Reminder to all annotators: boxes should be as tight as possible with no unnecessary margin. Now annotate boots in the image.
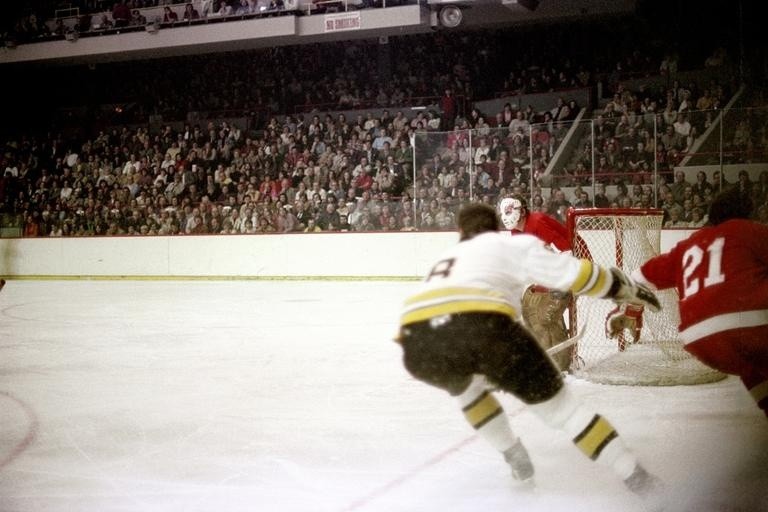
[622,464,665,499]
[502,436,535,481]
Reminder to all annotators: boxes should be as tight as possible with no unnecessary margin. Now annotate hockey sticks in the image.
[547,305,592,357]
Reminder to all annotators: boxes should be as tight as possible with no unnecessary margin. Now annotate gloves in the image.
[599,266,663,344]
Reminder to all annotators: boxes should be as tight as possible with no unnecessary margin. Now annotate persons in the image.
[602,181,767,424]
[391,201,667,503]
[1,1,767,233]
[494,188,600,375]
